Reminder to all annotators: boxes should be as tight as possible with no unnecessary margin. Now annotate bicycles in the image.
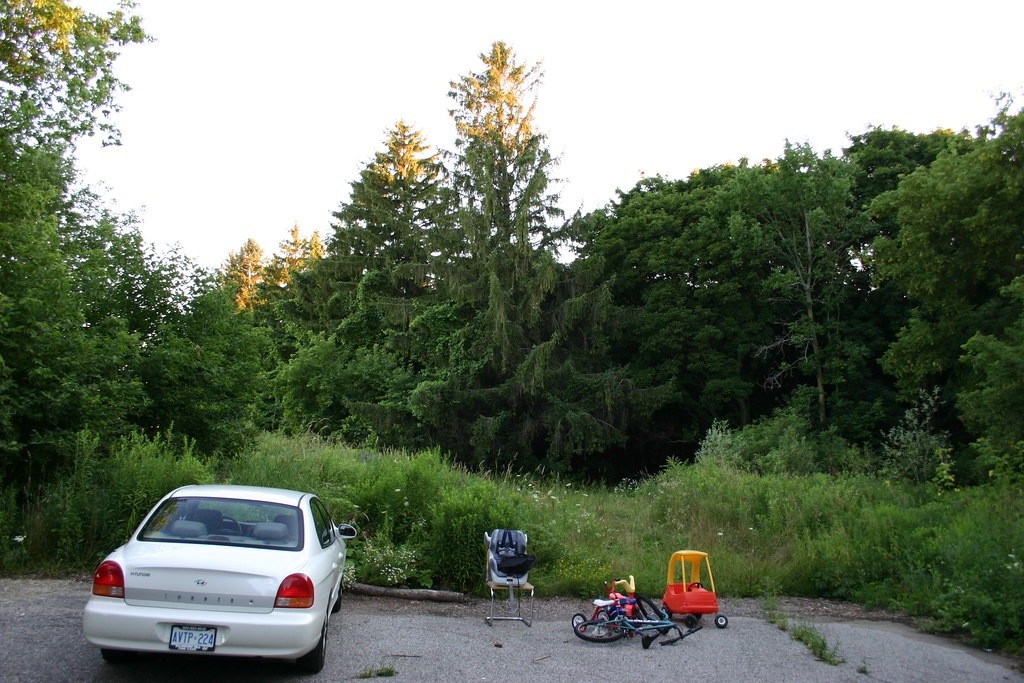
[572,574,704,649]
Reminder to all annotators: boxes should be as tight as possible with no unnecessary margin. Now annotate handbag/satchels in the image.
[496,553,537,578]
[496,529,518,557]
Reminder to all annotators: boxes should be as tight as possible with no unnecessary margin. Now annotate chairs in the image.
[171,520,207,537]
[278,515,297,537]
[196,510,223,531]
[255,521,285,541]
[485,529,534,627]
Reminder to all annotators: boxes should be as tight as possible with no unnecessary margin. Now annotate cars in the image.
[82,483,358,675]
